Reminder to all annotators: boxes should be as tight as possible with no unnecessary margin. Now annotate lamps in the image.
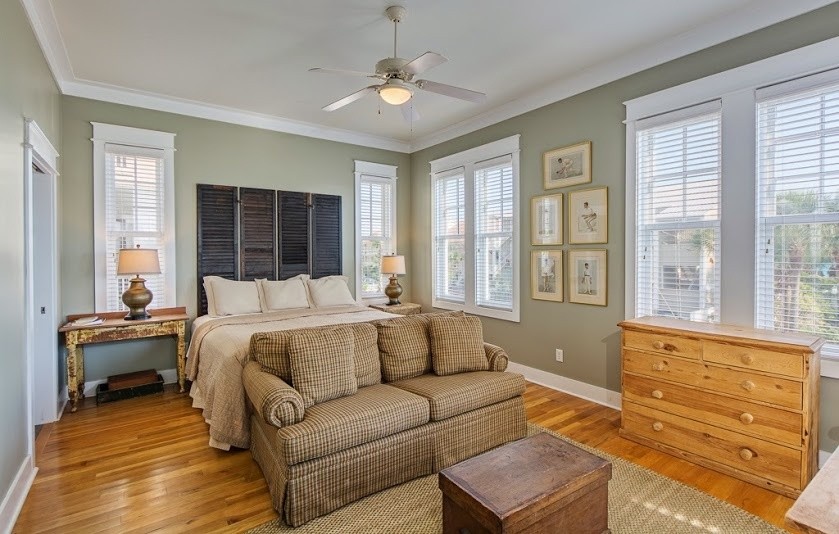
[117,244,161,320]
[380,252,406,306]
[376,80,415,137]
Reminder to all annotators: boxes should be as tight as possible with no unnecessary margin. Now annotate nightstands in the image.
[368,302,422,315]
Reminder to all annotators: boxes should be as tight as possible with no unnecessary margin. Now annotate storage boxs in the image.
[96,369,164,405]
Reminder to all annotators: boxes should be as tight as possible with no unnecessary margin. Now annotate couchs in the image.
[242,310,527,528]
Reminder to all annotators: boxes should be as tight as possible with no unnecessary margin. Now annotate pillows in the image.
[429,317,490,377]
[301,275,358,309]
[288,327,358,409]
[203,276,267,318]
[254,274,311,314]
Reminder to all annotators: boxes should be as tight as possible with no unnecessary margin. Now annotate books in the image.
[72,316,106,326]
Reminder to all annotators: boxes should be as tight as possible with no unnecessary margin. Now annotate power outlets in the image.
[556,349,563,363]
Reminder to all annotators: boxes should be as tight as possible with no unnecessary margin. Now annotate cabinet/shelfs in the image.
[616,314,826,499]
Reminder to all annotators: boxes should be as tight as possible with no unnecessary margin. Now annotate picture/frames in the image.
[543,141,592,191]
[567,248,608,307]
[530,193,564,246]
[529,249,564,303]
[568,185,608,245]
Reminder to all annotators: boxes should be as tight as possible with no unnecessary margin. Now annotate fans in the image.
[308,6,485,112]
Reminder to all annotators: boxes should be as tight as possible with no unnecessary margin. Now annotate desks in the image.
[58,307,189,413]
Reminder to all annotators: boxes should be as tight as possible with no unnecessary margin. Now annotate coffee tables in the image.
[439,432,613,534]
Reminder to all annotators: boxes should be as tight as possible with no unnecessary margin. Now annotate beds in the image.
[185,304,408,451]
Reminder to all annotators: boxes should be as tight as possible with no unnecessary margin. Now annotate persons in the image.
[555,157,574,179]
[580,200,597,232]
[541,259,552,292]
[581,263,593,294]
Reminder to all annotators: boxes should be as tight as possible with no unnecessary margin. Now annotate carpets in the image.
[243,420,793,534]
